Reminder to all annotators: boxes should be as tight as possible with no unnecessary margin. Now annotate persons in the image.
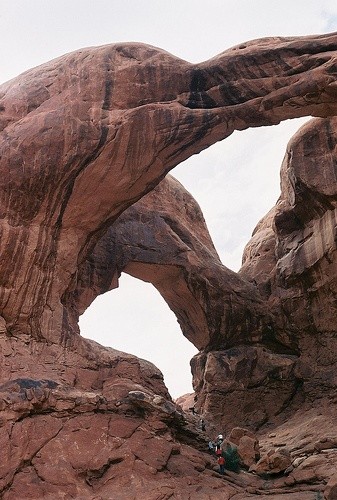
[217,432,223,442]
[253,440,263,463]
[214,442,223,473]
[227,442,239,460]
[208,437,214,455]
